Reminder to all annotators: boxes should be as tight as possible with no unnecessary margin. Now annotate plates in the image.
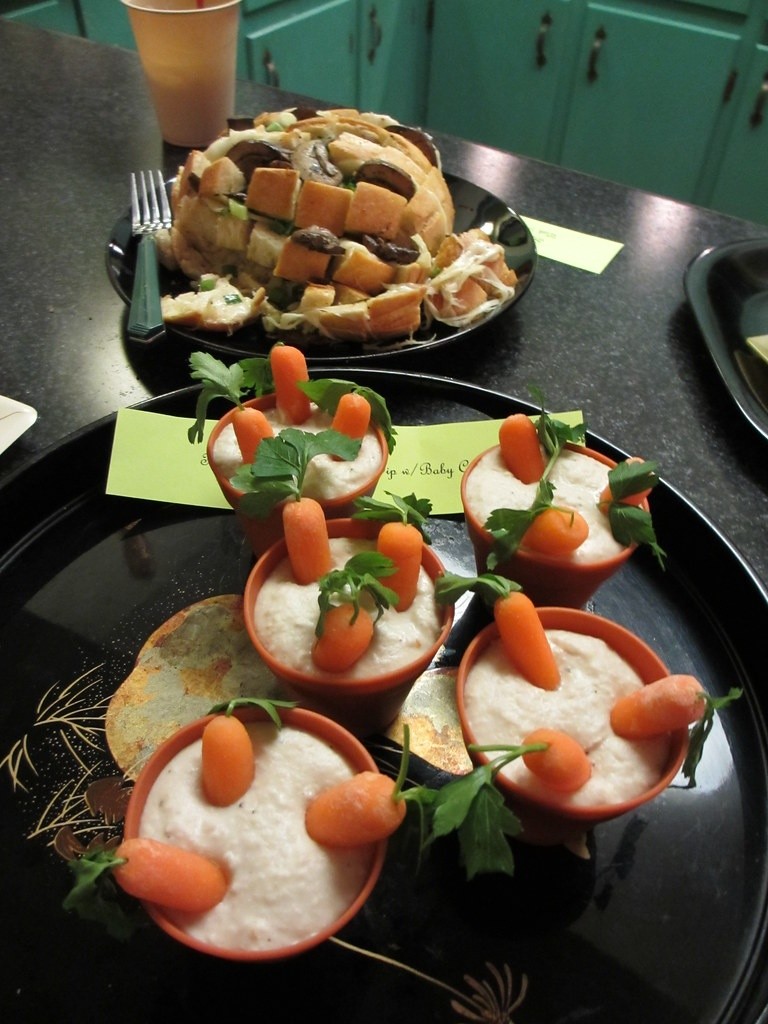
[683,235,768,441]
[105,171,538,364]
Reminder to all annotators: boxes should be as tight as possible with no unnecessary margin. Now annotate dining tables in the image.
[0,17,768,1024]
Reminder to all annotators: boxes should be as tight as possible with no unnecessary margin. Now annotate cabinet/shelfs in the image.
[424,0,752,203]
[709,0,768,225]
[240,0,430,127]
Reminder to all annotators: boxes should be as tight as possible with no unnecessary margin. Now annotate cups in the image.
[455,608,692,847]
[118,0,239,148]
[460,441,651,609]
[124,707,388,962]
[244,517,455,735]
[206,392,390,561]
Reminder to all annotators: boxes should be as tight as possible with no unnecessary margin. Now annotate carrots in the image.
[110,344,706,925]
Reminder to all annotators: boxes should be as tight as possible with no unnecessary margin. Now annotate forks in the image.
[125,173,172,342]
